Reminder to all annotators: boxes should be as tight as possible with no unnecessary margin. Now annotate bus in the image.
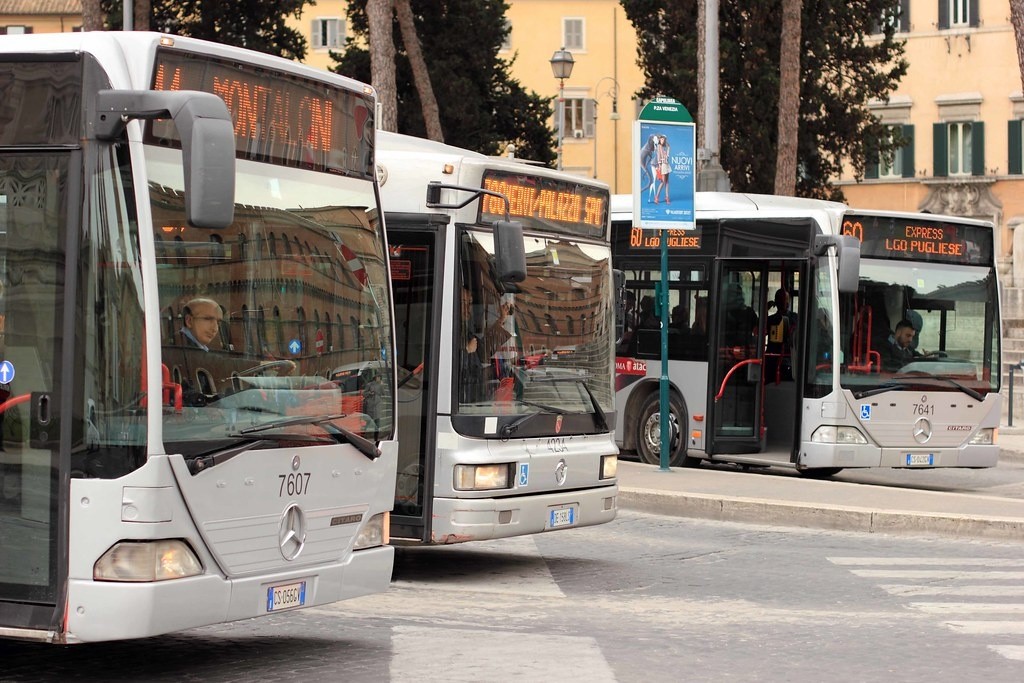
[374,130,619,549]
[610,192,1002,478]
[332,360,388,396]
[0,30,398,647]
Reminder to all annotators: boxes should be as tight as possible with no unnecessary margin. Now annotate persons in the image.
[624,290,709,355]
[875,319,931,371]
[161,299,295,389]
[647,135,671,205]
[462,290,512,380]
[906,309,924,350]
[755,288,799,373]
[726,282,759,348]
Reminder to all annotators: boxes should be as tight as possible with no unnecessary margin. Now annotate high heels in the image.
[665,196,670,204]
[655,195,658,203]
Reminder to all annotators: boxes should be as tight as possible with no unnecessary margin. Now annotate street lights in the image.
[593,77,620,178]
[551,47,574,169]
